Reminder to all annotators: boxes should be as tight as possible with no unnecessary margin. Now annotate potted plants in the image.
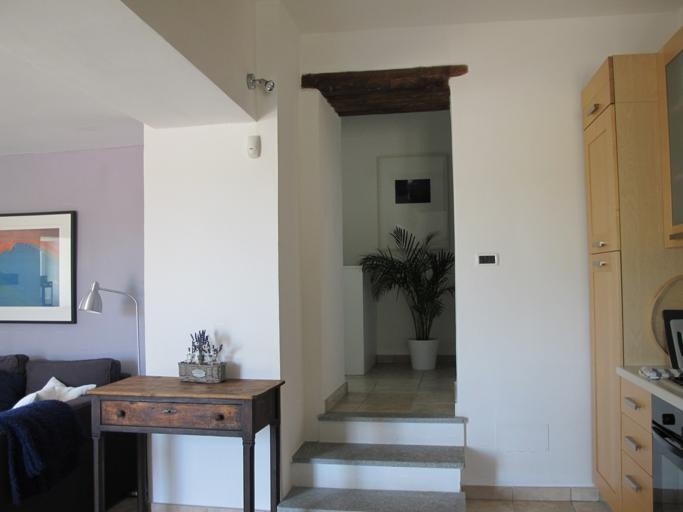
[361,224,455,372]
[177,329,229,385]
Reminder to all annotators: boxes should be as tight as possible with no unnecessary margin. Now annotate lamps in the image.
[80,276,144,377]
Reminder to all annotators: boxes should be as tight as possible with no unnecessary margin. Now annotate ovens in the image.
[652,425,683,512]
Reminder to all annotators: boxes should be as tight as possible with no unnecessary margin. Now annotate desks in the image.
[82,371,287,512]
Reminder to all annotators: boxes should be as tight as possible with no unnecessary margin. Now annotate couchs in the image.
[1,353,135,512]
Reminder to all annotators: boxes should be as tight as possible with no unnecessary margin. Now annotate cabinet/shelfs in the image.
[577,45,683,509]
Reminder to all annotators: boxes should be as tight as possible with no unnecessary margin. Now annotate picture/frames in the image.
[1,209,79,325]
